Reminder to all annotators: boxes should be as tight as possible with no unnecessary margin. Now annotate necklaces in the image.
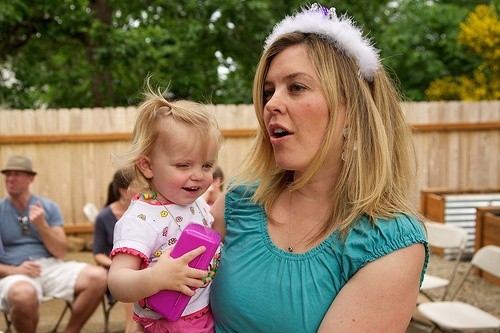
[287,188,327,253]
[142,192,217,283]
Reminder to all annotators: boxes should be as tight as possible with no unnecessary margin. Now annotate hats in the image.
[0,155,36,176]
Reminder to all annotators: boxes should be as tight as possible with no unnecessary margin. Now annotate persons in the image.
[94,167,145,333]
[108,71,224,333]
[0,156,108,333]
[210,2,429,333]
[202,167,224,205]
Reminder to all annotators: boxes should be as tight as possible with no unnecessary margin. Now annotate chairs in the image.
[0,291,118,333]
[411,221,500,333]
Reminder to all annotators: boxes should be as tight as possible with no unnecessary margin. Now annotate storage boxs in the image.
[147,224,222,322]
